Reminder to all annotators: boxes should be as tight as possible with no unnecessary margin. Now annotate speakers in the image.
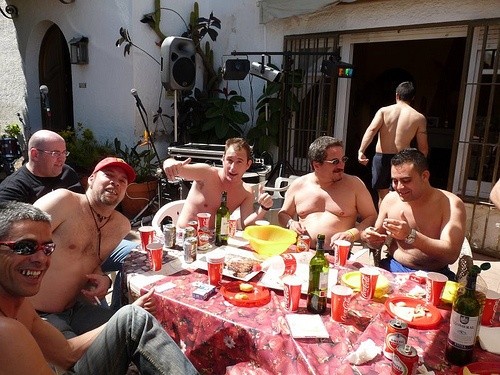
[160,36,196,91]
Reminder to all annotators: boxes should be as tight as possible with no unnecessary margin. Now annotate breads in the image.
[235,293,249,300]
[239,283,253,291]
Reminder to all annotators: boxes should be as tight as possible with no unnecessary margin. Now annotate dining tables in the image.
[119,229,500,375]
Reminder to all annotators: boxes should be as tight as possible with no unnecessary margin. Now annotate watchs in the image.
[405,229,417,245]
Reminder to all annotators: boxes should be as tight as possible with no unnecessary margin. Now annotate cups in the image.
[255,220,270,226]
[476,288,500,327]
[425,272,448,308]
[206,252,225,286]
[330,285,354,323]
[283,276,302,312]
[197,212,212,231]
[138,226,155,252]
[146,242,164,271]
[228,220,237,237]
[359,267,380,301]
[334,240,351,267]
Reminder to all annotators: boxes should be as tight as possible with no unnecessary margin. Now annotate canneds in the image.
[389,343,418,375]
[296,234,310,253]
[182,221,197,263]
[164,224,176,249]
[382,318,409,362]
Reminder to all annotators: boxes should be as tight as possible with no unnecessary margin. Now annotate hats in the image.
[92,157,136,186]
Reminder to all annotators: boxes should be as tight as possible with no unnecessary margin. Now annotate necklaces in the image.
[85,194,111,257]
[0,308,7,318]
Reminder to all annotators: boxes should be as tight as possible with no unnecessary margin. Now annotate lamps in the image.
[68,36,90,65]
[220,55,250,81]
[249,54,282,82]
[321,54,355,79]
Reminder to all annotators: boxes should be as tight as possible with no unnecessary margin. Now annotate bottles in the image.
[306,234,330,315]
[444,262,491,367]
[214,191,230,247]
[252,252,315,281]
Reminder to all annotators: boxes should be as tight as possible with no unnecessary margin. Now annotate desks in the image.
[241,164,273,187]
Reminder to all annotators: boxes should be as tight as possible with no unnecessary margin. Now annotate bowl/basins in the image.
[242,225,297,258]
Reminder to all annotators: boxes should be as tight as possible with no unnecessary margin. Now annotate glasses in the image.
[30,147,70,157]
[0,241,56,256]
[322,157,348,165]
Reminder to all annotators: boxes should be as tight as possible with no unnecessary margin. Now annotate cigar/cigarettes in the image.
[94,295,101,305]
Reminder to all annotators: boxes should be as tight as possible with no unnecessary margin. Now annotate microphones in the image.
[130,88,145,112]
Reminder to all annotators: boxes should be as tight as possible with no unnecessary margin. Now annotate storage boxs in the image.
[167,142,226,168]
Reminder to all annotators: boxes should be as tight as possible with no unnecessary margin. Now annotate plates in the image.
[384,297,443,327]
[341,271,390,291]
[198,245,262,282]
[257,262,338,299]
[220,281,271,307]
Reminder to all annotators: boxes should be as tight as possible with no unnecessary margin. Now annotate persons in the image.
[278,136,377,260]
[27,158,137,340]
[0,130,84,205]
[0,200,202,375]
[163,137,273,233]
[490,178,500,211]
[360,148,466,282]
[358,81,428,210]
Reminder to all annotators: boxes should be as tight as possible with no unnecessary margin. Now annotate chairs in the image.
[255,175,299,229]
[151,199,187,237]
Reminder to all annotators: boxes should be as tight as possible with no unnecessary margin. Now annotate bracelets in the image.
[346,230,355,242]
[286,220,293,228]
[260,203,271,211]
